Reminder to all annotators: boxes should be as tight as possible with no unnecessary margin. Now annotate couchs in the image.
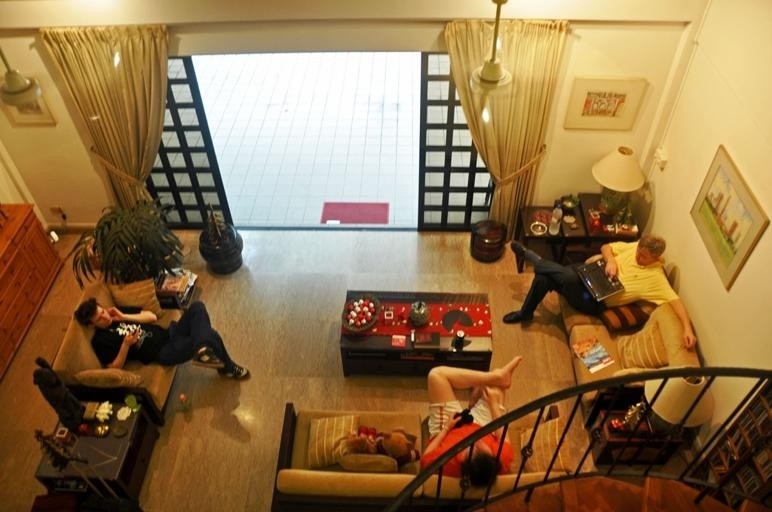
[270,402,568,512]
[558,254,701,418]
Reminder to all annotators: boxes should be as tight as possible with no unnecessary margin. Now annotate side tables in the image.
[589,410,684,466]
[34,402,160,504]
[157,267,200,312]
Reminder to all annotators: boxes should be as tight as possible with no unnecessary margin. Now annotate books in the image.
[160,269,199,304]
[412,330,442,350]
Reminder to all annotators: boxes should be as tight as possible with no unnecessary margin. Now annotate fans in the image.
[470,0,513,95]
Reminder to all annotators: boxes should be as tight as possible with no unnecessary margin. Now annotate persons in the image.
[585,94,625,117]
[497,229,699,352]
[72,297,252,381]
[416,353,526,489]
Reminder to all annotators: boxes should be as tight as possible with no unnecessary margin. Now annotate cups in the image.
[125,395,138,409]
[454,330,465,351]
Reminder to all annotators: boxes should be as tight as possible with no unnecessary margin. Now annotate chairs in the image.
[52,271,184,426]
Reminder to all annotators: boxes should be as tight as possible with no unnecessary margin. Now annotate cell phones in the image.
[133,325,140,335]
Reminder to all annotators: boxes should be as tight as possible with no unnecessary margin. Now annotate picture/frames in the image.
[563,76,649,131]
[0,76,56,128]
[689,144,770,291]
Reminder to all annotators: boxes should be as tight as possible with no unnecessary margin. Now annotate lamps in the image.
[592,144,645,215]
[0,47,41,106]
[644,366,715,433]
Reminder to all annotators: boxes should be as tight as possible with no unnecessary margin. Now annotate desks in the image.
[514,193,641,273]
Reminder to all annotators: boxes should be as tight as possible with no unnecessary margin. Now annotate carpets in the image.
[320,202,389,224]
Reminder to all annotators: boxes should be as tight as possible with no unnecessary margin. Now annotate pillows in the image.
[306,414,398,473]
[616,320,670,370]
[75,368,144,387]
[520,417,564,473]
[107,277,163,319]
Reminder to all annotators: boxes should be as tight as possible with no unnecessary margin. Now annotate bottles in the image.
[548,204,564,236]
[179,393,193,418]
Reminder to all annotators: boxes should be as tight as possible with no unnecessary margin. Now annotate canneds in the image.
[454,329,465,348]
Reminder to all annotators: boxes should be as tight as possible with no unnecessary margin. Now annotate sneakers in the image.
[192,345,224,369]
[511,241,527,256]
[218,363,249,378]
[503,311,533,323]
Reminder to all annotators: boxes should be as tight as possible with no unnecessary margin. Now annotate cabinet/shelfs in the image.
[0,203,64,382]
[679,375,772,510]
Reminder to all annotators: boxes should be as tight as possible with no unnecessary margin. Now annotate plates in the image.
[341,294,382,334]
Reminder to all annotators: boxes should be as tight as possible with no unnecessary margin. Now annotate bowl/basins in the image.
[531,222,547,237]
[561,194,580,211]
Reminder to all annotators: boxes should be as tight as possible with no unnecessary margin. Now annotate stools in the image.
[470,220,507,263]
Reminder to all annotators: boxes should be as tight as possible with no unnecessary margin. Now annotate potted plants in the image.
[199,203,243,275]
[67,199,184,290]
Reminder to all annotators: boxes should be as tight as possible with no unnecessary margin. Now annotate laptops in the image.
[568,258,625,303]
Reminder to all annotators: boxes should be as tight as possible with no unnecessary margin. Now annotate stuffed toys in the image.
[375,427,422,465]
[343,424,380,453]
